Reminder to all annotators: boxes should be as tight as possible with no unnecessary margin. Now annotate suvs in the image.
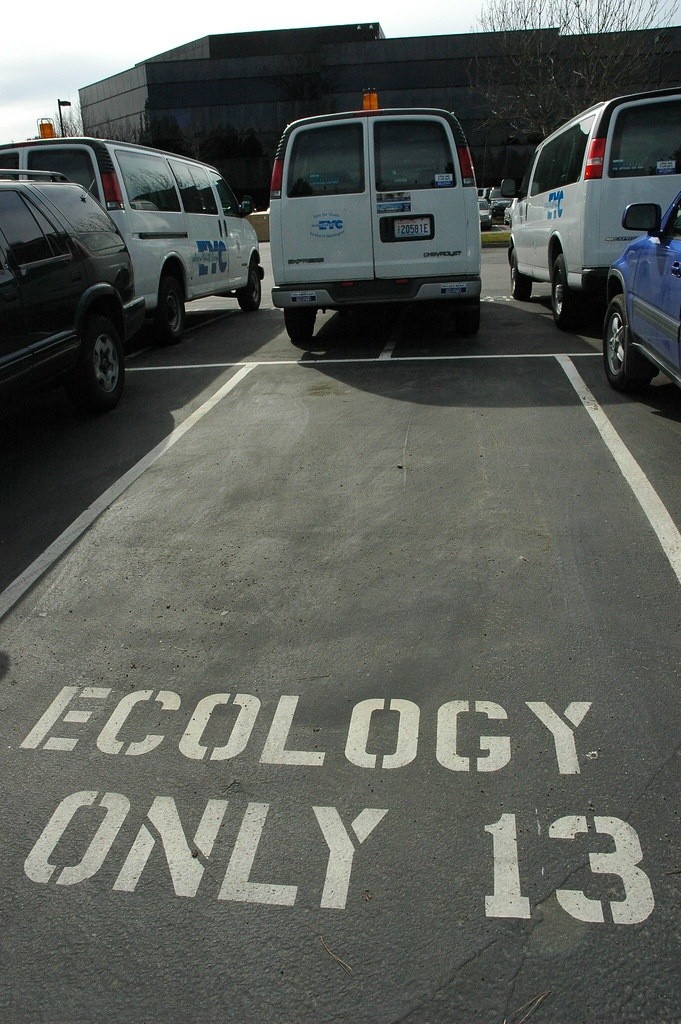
[478,188,511,216]
[0,170,148,422]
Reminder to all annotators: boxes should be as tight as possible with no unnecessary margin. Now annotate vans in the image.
[0,137,265,346]
[500,86,681,332]
[269,108,482,347]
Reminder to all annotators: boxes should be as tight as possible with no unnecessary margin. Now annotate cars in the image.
[605,190,681,396]
[478,199,492,229]
[503,198,518,226]
